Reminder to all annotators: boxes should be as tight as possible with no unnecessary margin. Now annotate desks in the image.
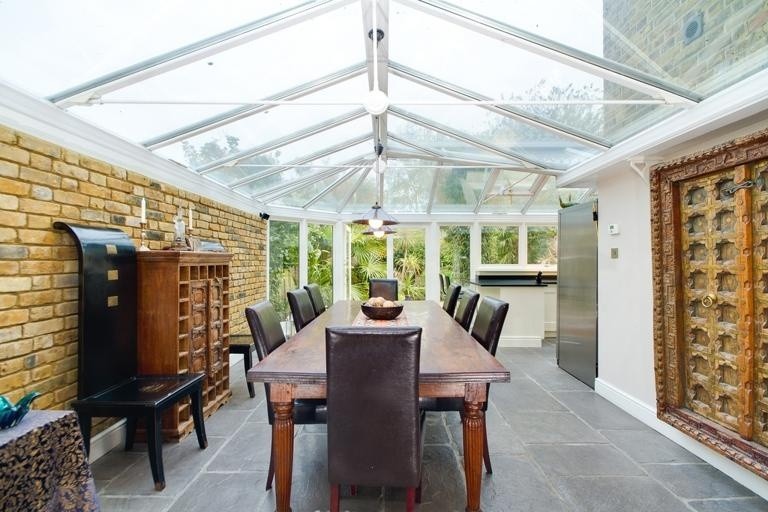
[0,405,99,512]
[247,300,512,512]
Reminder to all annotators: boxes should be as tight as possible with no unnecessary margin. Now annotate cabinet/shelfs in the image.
[542,287,558,338]
[135,249,235,445]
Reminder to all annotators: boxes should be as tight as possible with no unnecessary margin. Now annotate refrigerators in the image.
[556,198,597,390]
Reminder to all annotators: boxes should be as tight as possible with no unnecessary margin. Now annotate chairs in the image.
[52,220,210,492]
[454,287,481,332]
[366,277,400,302]
[287,288,316,332]
[195,239,258,398]
[442,281,461,318]
[419,296,511,475]
[303,282,327,314]
[244,300,327,491]
[322,324,426,512]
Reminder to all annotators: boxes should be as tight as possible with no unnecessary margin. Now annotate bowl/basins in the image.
[362,302,403,321]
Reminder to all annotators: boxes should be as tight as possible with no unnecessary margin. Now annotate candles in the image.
[188,209,194,231]
[140,198,147,222]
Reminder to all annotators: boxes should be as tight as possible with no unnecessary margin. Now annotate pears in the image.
[365,297,402,307]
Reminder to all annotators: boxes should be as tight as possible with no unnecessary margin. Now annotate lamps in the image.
[361,148,398,238]
[352,115,400,228]
